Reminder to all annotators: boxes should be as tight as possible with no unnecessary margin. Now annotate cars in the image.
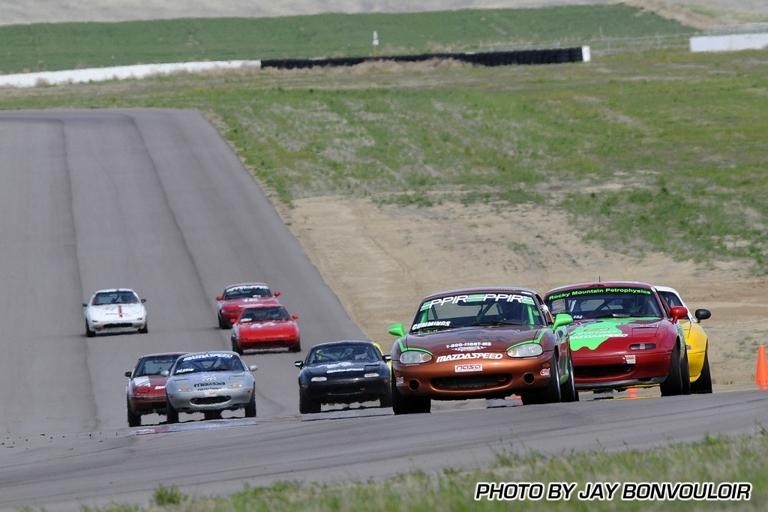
[388,287,576,415]
[295,340,393,413]
[651,285,712,393]
[544,282,690,402]
[216,282,280,330]
[82,289,148,337]
[165,351,257,423]
[124,352,190,425]
[231,305,300,355]
